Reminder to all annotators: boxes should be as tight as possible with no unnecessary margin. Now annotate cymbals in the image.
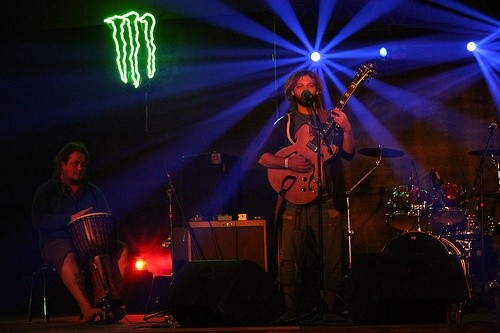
[431,181,467,226]
[384,184,424,232]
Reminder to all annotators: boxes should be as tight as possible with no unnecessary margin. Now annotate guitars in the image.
[267,60,378,205]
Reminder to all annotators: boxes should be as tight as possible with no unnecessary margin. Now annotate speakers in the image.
[118,152,285,331]
[367,296,448,325]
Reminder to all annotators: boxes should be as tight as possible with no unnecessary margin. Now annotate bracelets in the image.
[285,158,289,168]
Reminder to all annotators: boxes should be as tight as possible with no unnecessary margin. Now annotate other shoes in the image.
[323,311,355,325]
[268,312,300,326]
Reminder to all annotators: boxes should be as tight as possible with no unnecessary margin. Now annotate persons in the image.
[257,70,356,327]
[32,142,128,322]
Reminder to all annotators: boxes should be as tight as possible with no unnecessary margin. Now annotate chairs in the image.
[27,228,91,323]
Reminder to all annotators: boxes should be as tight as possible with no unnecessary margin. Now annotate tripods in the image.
[281,98,352,327]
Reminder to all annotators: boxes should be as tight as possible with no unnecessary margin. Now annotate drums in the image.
[67,213,126,324]
[376,231,466,322]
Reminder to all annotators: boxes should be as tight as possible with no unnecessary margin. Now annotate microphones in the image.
[302,90,313,100]
[432,167,444,183]
[489,122,498,130]
[408,166,414,187]
